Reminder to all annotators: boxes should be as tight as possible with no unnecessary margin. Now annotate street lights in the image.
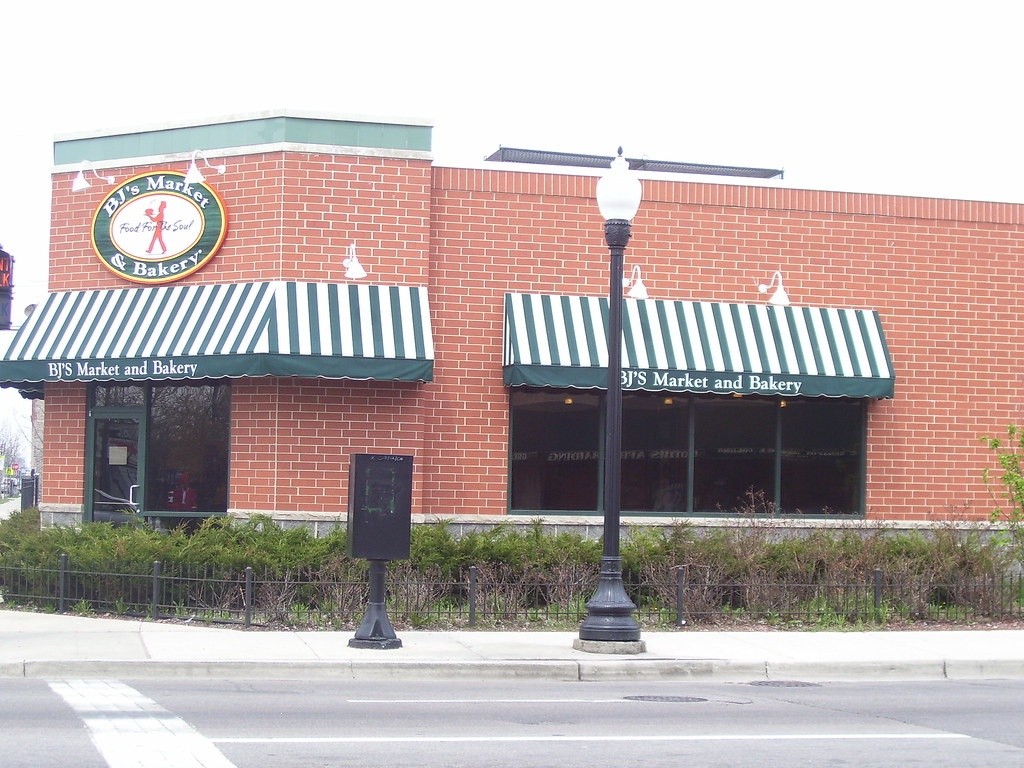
[571,150,647,656]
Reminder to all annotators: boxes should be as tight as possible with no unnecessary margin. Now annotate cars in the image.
[1,477,18,493]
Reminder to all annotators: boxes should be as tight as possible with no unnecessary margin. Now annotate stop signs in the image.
[12,464,18,470]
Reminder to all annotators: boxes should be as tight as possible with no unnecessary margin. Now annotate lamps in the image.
[343,243,368,279]
[184,149,227,183]
[758,272,792,307]
[72,160,115,192]
[623,264,650,300]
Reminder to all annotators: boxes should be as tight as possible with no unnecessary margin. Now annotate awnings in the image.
[0,281,435,388]
[502,292,895,400]
[18,389,46,400]
